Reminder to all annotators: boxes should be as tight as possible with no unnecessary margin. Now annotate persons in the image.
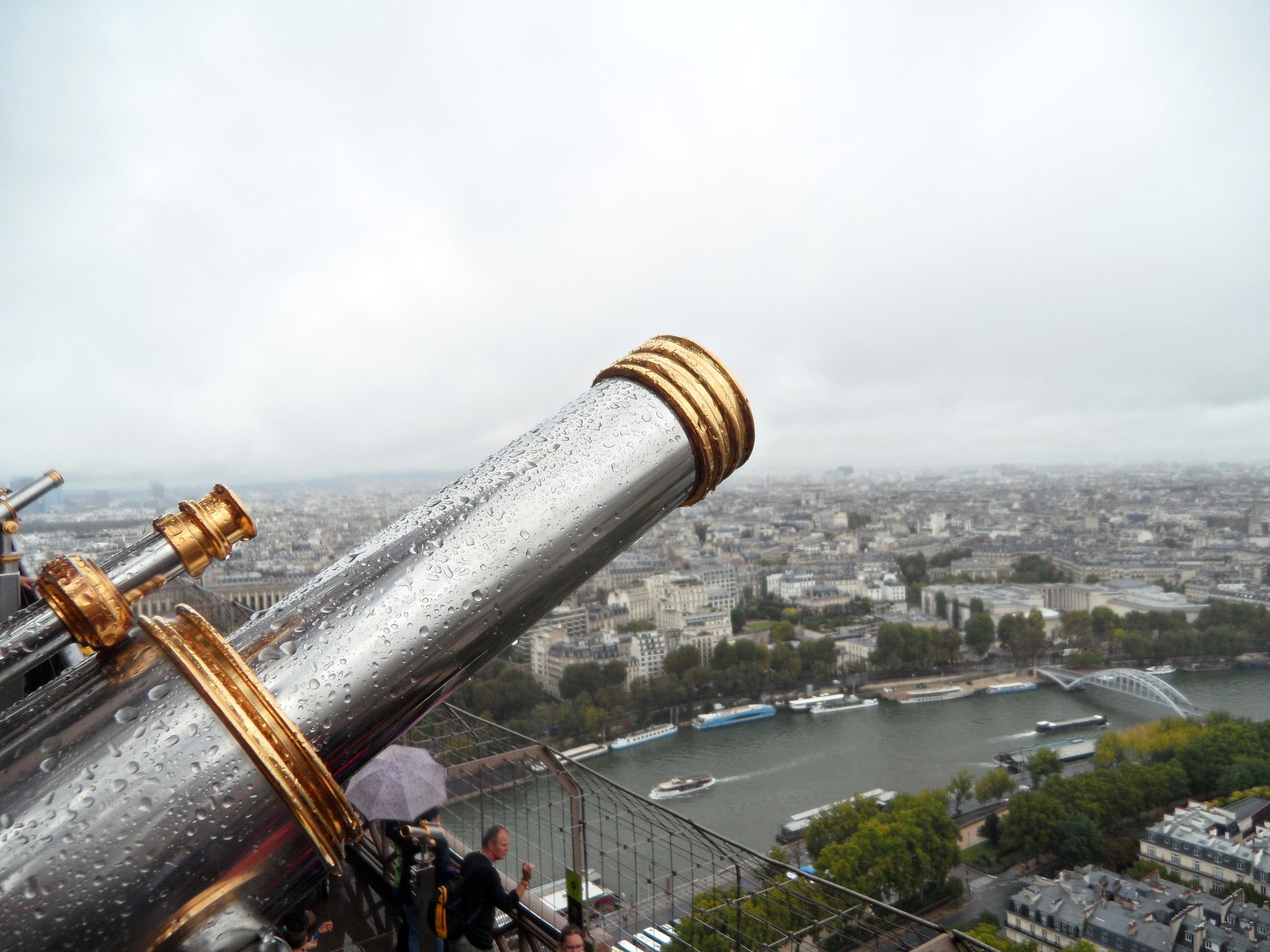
[283,803,447,952]
[448,825,535,952]
[557,923,585,952]
[19,574,37,591]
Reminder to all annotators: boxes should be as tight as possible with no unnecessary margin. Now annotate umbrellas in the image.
[346,744,448,822]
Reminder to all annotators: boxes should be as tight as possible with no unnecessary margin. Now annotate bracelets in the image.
[521,877,530,883]
[519,880,528,889]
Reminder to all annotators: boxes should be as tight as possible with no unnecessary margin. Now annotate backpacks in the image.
[427,860,489,941]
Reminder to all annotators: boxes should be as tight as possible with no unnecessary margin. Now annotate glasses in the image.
[563,943,586,952]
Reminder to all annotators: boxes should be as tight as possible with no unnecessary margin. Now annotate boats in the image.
[527,647,1109,924]
[1144,652,1270,675]
